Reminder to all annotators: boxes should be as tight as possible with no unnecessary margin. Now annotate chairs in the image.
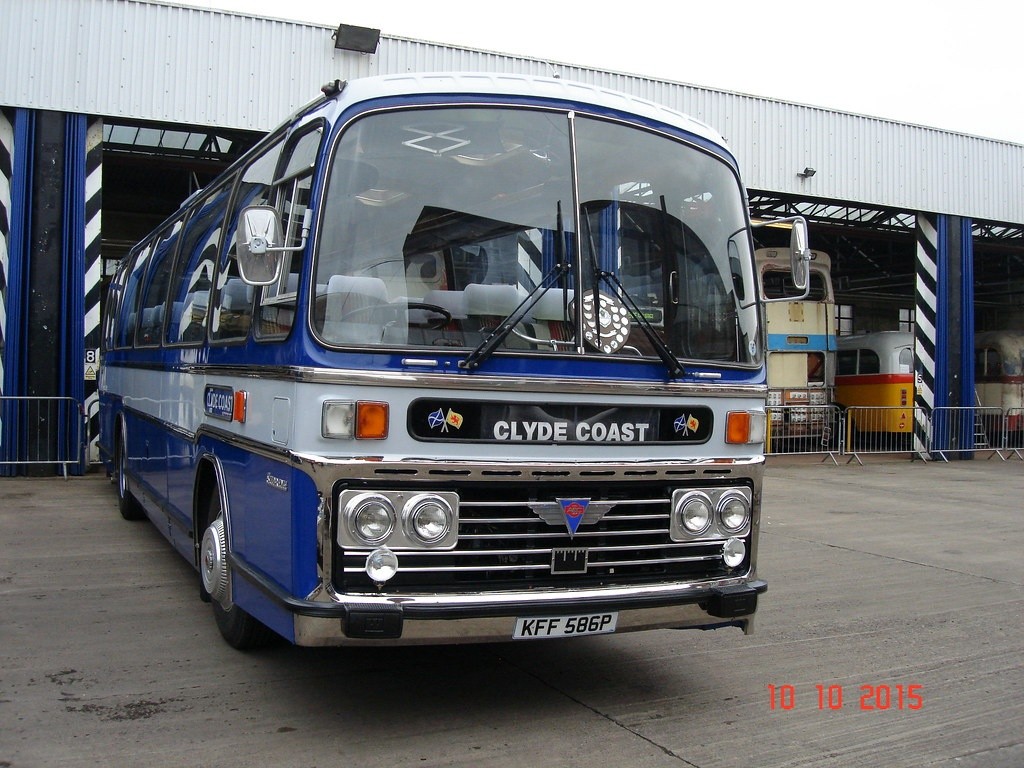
[125,272,575,353]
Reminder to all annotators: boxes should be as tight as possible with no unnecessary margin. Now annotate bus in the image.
[835,330,915,446]
[619,246,836,441]
[974,327,1024,448]
[94,74,813,654]
